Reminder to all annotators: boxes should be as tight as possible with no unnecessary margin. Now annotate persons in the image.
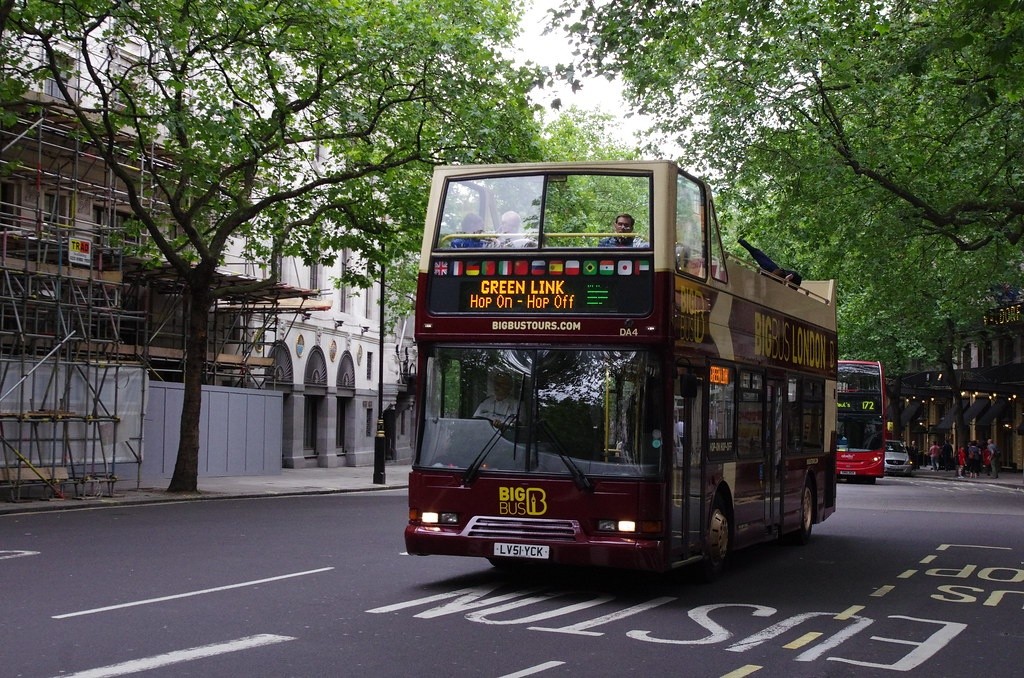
[598,214,649,247]
[905,439,1000,478]
[450,211,538,248]
[738,235,802,291]
[473,373,525,431]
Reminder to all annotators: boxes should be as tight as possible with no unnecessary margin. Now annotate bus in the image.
[833,359,888,484]
[405,159,842,584]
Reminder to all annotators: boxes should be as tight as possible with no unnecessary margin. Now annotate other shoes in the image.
[992,476,998,479]
[958,476,964,479]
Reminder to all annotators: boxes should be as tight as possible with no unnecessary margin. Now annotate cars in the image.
[884,440,912,477]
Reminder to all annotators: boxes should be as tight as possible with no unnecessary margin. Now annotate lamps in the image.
[335,320,344,327]
[301,312,313,322]
[362,326,370,334]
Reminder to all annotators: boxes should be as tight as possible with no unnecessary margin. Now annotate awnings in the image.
[887,397,1008,429]
